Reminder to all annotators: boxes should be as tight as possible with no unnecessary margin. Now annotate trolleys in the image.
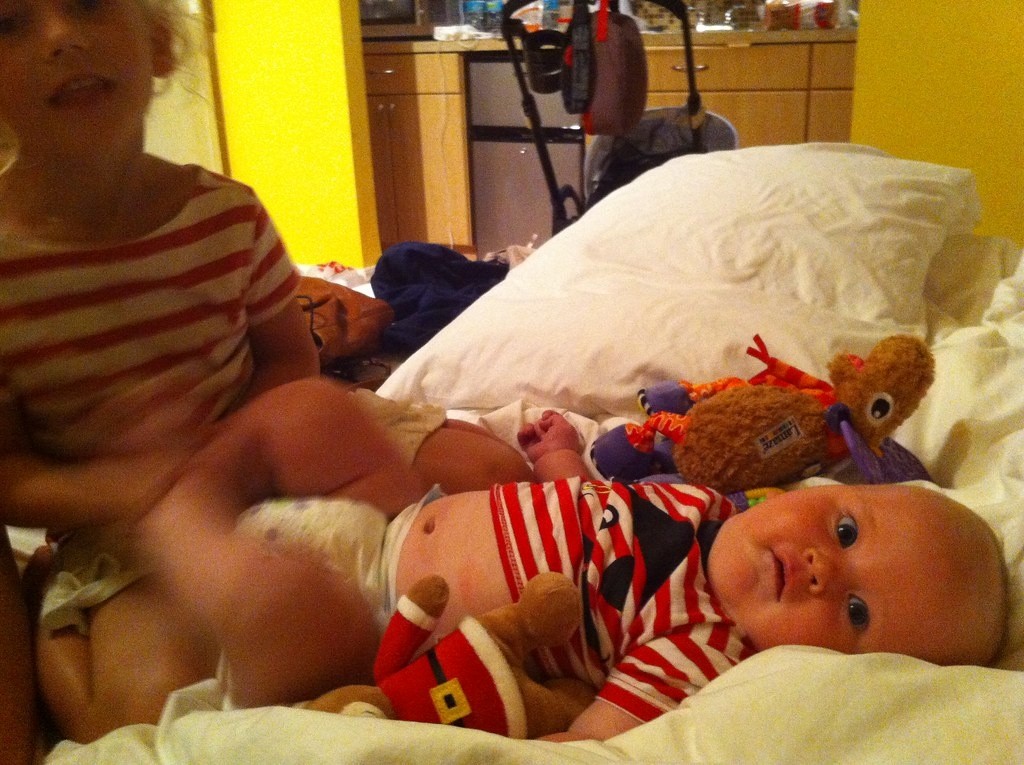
[496,2,739,228]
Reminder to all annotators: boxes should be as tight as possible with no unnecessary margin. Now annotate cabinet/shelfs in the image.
[622,40,856,175]
[361,52,481,266]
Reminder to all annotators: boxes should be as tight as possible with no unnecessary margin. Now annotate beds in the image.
[0,238,1024,762]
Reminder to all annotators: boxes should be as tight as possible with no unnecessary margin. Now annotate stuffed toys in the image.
[303,573,593,739]
[595,333,942,514]
[287,240,537,366]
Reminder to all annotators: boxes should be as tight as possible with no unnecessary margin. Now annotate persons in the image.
[2,3,327,740]
[86,368,1011,741]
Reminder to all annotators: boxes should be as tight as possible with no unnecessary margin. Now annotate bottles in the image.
[542,1,558,31]
[463,0,486,33]
[485,0,503,42]
